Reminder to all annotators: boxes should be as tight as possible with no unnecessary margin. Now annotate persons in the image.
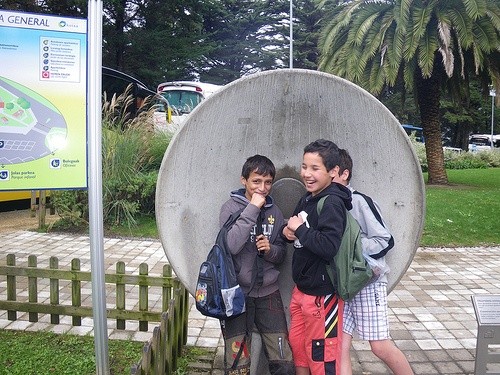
[218,155,295,375]
[276,139,353,375]
[330,148,414,374]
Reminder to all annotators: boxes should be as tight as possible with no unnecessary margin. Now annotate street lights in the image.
[487,80,497,149]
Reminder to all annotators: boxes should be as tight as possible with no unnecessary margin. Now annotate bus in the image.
[153,80,225,136]
[102,65,171,137]
[401,124,427,171]
[467,134,500,154]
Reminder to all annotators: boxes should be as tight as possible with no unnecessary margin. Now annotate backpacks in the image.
[307,193,375,303]
[195,204,265,326]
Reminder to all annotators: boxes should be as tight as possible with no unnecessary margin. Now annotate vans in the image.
[442,146,464,155]
